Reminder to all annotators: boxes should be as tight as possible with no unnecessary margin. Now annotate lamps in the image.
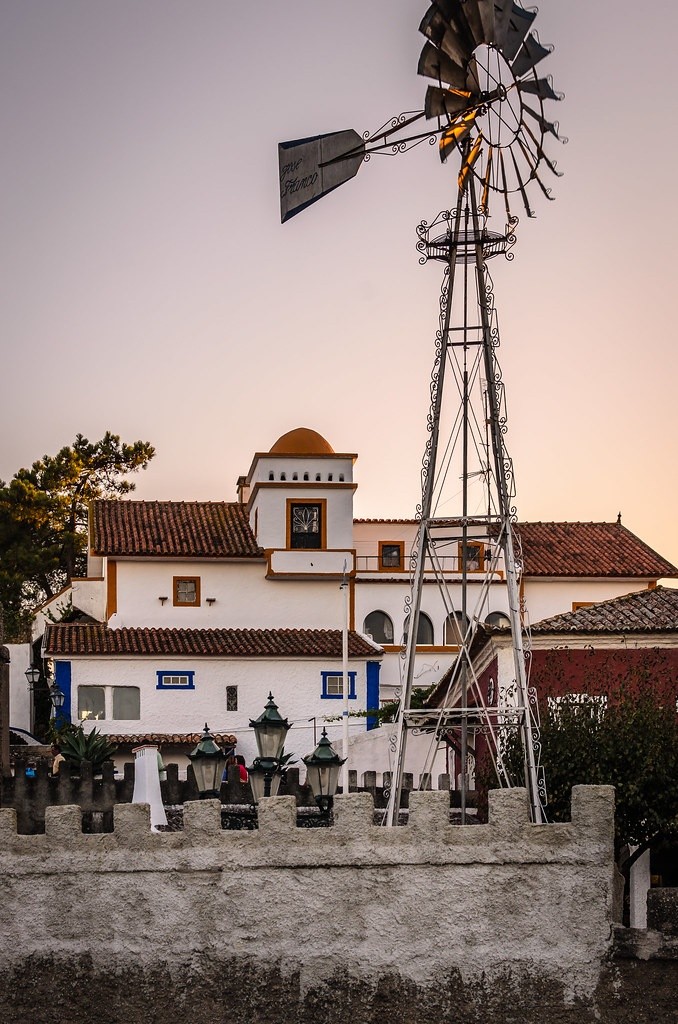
[50,684,70,721]
[25,662,50,691]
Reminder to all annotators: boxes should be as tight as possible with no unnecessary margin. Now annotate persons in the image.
[234,755,249,804]
[220,759,232,804]
[49,746,65,778]
